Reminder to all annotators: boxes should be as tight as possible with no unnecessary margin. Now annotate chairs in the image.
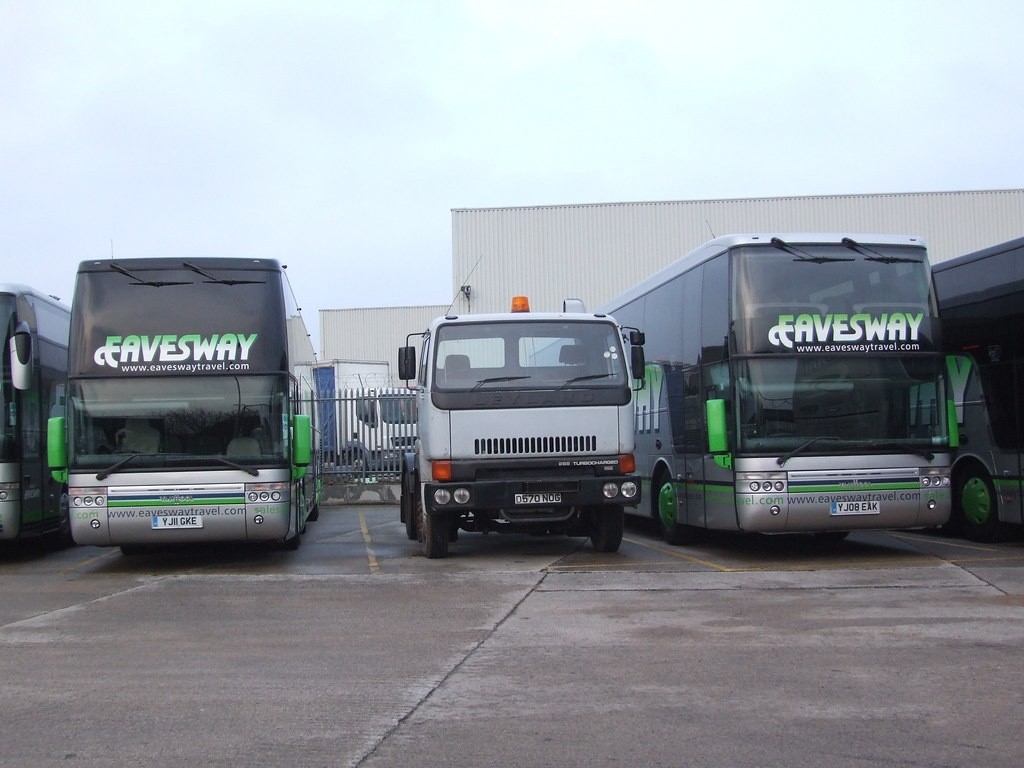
[114,420,160,453]
[226,436,262,455]
[438,353,480,388]
[559,343,591,380]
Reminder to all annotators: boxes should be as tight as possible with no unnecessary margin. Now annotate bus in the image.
[842,235,1024,543]
[0,282,116,550]
[525,230,962,547]
[46,257,328,556]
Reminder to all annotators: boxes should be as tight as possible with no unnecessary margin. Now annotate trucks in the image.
[293,358,418,481]
[399,295,648,559]
